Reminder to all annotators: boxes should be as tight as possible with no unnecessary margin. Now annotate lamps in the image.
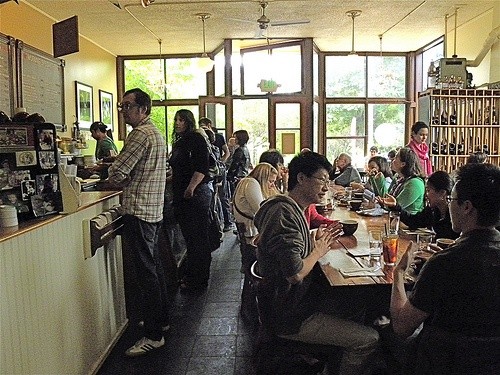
[191,12,221,72]
[345,8,362,57]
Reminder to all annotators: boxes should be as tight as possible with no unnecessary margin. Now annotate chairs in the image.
[235,222,342,375]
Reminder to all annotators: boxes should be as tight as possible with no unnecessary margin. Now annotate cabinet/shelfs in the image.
[418,86,500,175]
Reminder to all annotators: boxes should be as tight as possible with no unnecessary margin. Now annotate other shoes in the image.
[181,281,207,290]
[222,226,234,231]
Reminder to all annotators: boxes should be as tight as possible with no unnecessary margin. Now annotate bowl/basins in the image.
[339,219,358,236]
[316,204,325,211]
[437,238,455,248]
[340,192,364,208]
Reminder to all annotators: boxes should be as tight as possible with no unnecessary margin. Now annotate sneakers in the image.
[124,337,165,357]
[139,322,169,331]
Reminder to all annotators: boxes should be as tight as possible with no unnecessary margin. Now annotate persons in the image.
[404,121,432,176]
[196,118,234,232]
[162,109,217,293]
[233,162,278,250]
[228,130,252,234]
[303,202,341,230]
[468,152,490,166]
[375,149,425,216]
[97,89,173,356]
[370,146,378,158]
[260,149,289,198]
[385,164,500,375]
[201,126,224,250]
[388,145,403,161]
[90,122,118,164]
[329,153,362,185]
[374,171,460,242]
[365,157,393,198]
[301,148,311,153]
[251,152,381,375]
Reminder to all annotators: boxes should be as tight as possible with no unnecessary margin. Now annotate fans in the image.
[228,0,310,39]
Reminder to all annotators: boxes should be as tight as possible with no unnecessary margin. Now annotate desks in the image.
[308,181,437,327]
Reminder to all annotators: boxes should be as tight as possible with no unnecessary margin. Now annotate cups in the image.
[382,233,399,266]
[389,211,402,234]
[0,205,19,227]
[417,233,432,252]
[369,230,383,260]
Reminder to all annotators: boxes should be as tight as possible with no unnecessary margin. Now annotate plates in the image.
[413,251,432,259]
[338,197,389,215]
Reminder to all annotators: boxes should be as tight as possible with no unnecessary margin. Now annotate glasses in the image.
[118,102,140,111]
[305,173,330,186]
[446,196,465,203]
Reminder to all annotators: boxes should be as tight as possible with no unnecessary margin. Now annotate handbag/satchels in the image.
[189,134,220,183]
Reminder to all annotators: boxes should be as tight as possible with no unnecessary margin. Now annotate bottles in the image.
[60,158,77,191]
[75,154,85,168]
[84,154,95,169]
[427,62,436,89]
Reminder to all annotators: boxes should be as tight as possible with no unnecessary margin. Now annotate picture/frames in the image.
[74,80,94,129]
[99,89,115,132]
